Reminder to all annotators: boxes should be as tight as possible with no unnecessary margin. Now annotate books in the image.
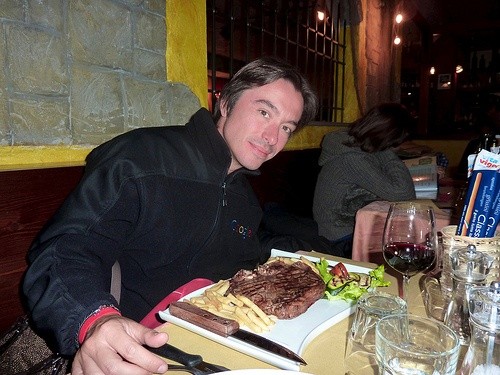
[402,156,438,200]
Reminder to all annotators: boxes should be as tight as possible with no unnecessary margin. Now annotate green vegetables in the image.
[316,260,391,302]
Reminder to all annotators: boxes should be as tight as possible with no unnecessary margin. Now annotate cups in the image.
[342,292,408,375]
[423,238,471,321]
[374,313,460,375]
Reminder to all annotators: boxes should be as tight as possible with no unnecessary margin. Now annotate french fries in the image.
[182,281,278,332]
[264,256,325,274]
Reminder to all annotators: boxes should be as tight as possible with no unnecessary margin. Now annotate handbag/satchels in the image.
[0,312,70,375]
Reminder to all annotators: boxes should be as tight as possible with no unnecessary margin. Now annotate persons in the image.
[313,103,416,259]
[20,58,319,375]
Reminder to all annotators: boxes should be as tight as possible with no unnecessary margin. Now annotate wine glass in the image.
[382,201,438,304]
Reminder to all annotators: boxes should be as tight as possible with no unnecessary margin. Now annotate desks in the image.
[353,198,500,263]
[145,250,500,375]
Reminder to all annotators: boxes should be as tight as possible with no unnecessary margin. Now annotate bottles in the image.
[439,153,449,179]
[443,244,500,375]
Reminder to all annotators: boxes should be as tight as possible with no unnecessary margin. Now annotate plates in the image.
[159,247,377,371]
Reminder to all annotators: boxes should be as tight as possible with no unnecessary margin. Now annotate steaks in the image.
[228,260,327,320]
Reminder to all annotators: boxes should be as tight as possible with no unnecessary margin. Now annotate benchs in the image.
[0,147,321,341]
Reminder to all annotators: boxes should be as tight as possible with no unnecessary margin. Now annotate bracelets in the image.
[78,306,122,344]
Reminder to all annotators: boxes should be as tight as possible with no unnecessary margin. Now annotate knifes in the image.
[143,342,231,373]
[169,301,308,366]
